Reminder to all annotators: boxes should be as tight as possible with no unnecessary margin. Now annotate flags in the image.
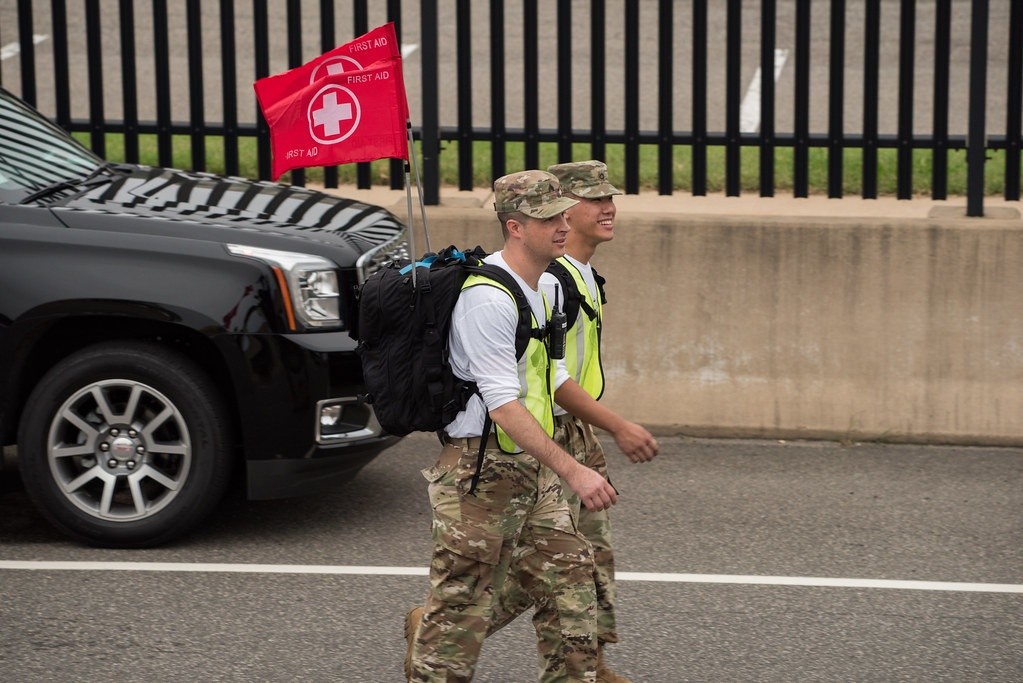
[267,55,411,182]
[252,22,413,131]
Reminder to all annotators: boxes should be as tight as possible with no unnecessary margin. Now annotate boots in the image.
[404,606,426,683]
[596,640,634,683]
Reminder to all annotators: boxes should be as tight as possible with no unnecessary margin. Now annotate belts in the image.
[447,435,500,450]
[553,413,576,427]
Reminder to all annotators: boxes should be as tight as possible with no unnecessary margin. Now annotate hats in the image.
[547,160,623,199]
[493,169,580,219]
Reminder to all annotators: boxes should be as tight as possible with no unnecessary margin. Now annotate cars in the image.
[0,84,414,551]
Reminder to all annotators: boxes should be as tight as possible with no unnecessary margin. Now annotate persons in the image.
[410,169,617,683]
[403,160,660,681]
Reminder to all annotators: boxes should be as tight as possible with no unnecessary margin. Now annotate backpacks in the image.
[354,245,532,438]
